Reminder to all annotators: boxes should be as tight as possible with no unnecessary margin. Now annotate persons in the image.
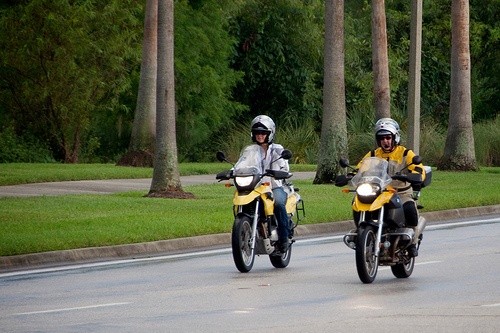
[216,115,289,253]
[335,117,425,258]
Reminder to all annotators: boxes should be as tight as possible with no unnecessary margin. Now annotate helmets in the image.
[249,114,276,144]
[374,117,401,146]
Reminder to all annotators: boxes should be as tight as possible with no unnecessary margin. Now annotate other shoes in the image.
[408,244,417,258]
[278,238,288,253]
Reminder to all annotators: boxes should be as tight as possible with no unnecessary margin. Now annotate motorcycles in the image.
[216,145,305,274]
[336,154,432,284]
